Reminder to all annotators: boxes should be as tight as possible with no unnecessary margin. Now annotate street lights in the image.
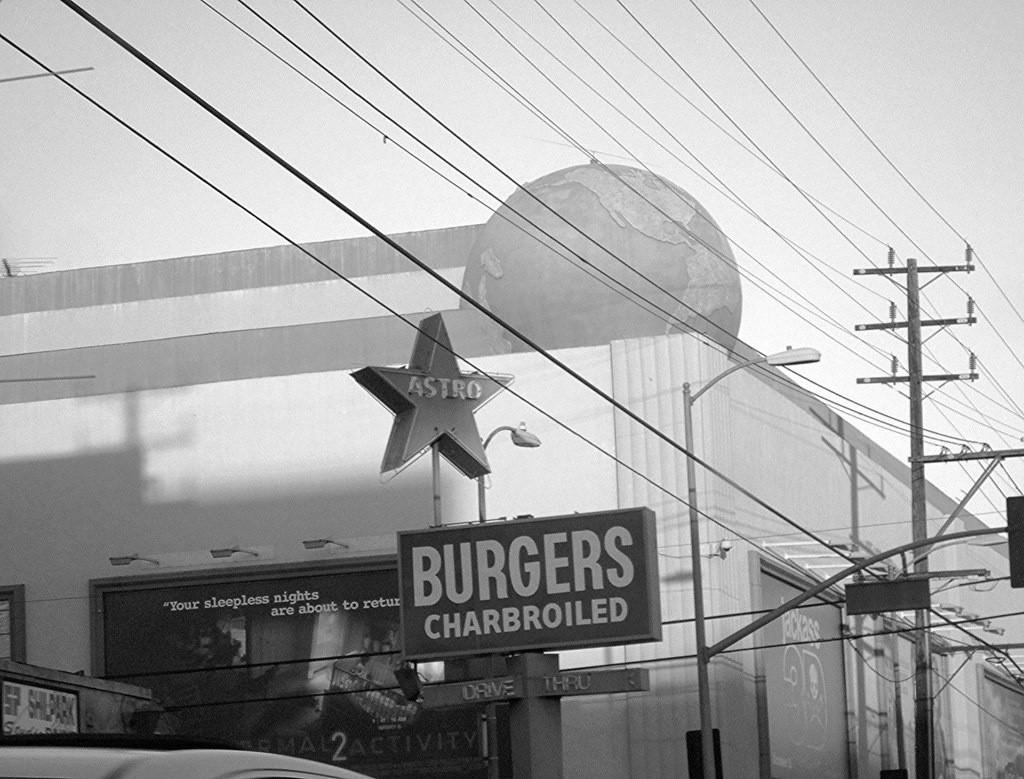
[478,420,541,779]
[682,345,822,779]
